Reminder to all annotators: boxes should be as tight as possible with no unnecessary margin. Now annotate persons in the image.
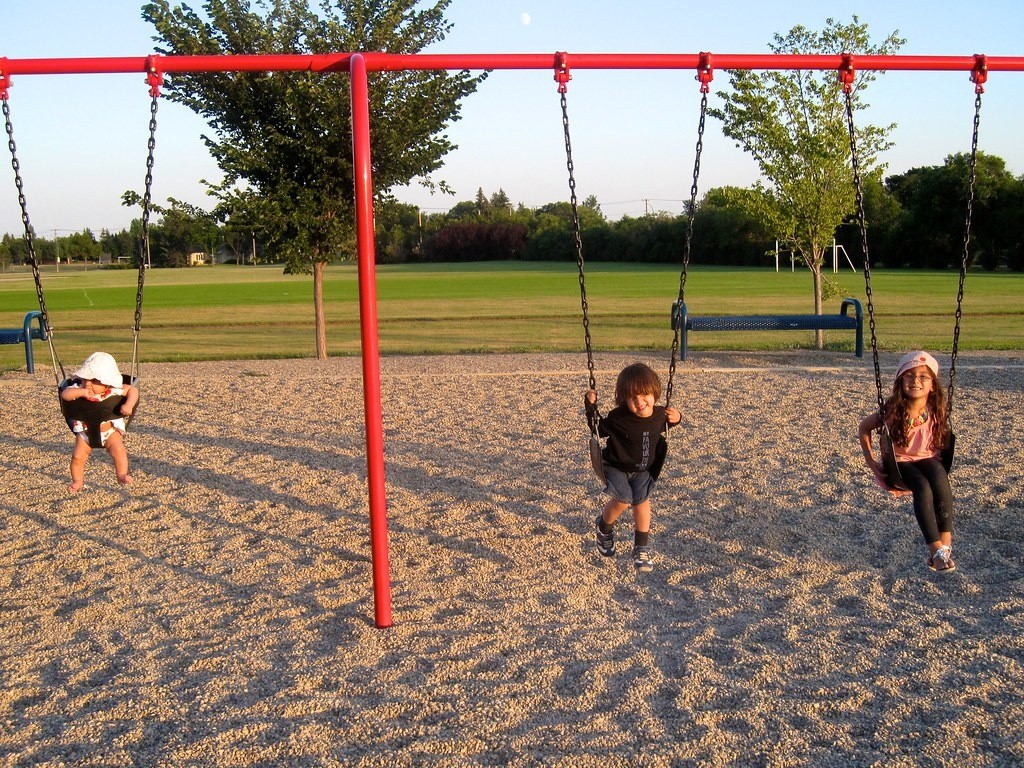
[60,352,133,491]
[584,363,682,572]
[859,350,956,574]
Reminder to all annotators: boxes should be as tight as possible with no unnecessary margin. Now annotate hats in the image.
[71,352,122,389]
[894,351,939,380]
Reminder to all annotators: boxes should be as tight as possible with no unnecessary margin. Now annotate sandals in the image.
[925,545,954,574]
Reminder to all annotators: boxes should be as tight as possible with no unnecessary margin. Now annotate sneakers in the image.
[633,545,653,572]
[596,515,616,557]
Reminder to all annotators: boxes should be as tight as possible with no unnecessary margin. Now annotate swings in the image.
[557,69,714,486]
[839,75,980,494]
[1,91,160,445]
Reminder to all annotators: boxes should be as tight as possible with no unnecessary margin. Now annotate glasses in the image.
[900,372,935,383]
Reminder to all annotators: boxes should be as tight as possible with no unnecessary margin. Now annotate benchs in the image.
[0,311,48,374]
[670,298,864,358]
